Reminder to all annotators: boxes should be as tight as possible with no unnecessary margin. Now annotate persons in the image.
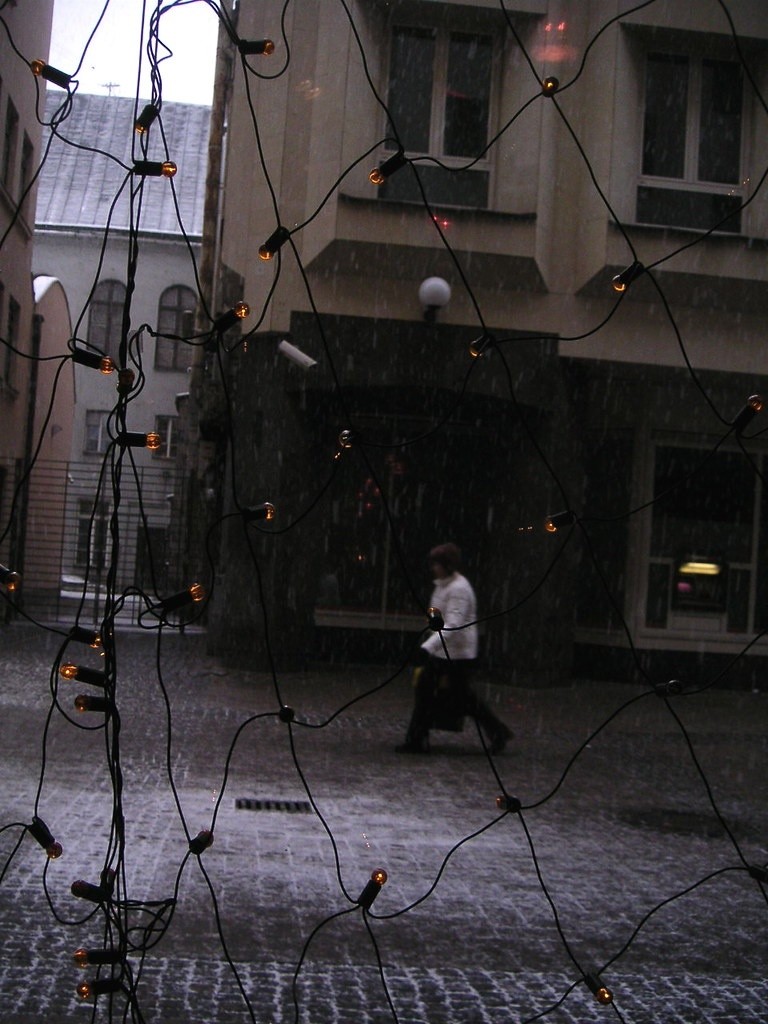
[394,543,516,755]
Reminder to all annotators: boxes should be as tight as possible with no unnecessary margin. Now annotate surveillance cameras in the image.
[278,339,317,375]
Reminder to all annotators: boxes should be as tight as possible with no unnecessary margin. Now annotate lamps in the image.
[417,275,453,329]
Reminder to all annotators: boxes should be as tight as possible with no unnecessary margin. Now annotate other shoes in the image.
[488,726,516,756]
[397,739,432,751]
